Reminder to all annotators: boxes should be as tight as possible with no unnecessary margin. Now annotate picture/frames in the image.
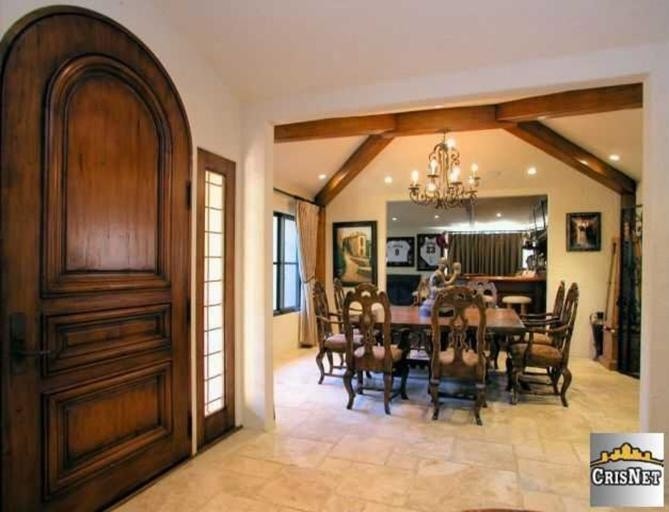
[331,220,379,287]
[565,210,603,253]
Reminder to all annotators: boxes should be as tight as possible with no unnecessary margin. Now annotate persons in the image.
[429,256,461,302]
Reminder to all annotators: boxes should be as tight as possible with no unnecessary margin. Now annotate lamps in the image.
[405,131,482,211]
